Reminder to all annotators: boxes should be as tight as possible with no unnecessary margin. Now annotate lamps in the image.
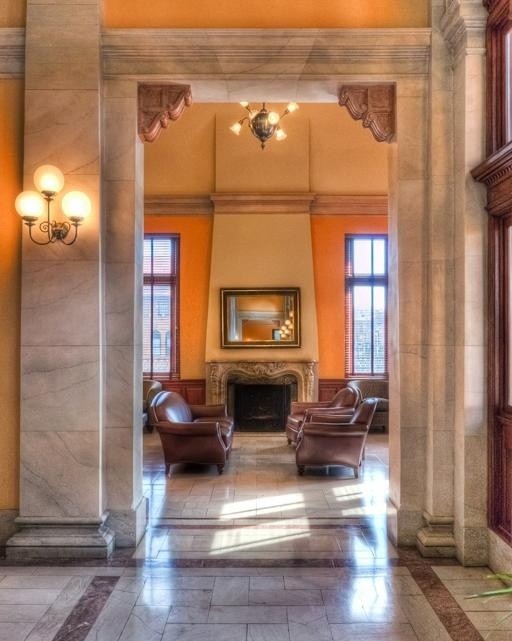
[13,164,94,247]
[229,102,299,149]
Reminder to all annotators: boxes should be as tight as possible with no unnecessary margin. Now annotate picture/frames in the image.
[217,288,306,351]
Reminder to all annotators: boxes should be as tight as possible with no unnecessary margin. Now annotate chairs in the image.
[144,380,236,480]
[287,379,390,479]
